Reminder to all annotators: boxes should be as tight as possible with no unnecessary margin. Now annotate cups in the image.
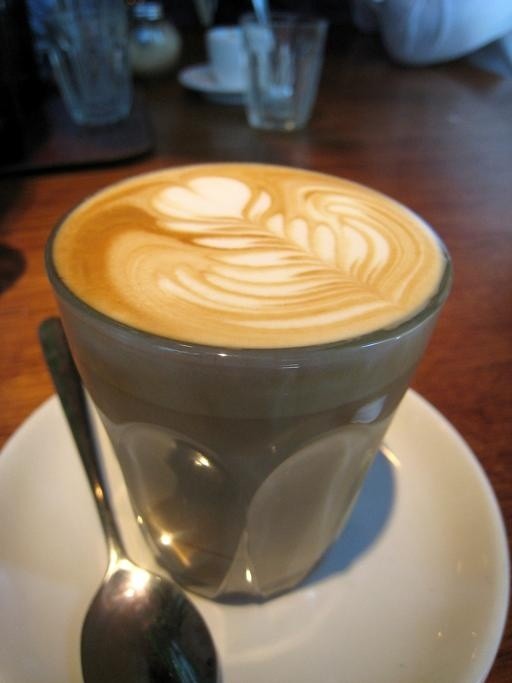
[243,16,327,129]
[43,162,452,601]
[39,0,132,126]
[205,27,273,86]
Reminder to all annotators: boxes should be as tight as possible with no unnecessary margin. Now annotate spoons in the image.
[33,316,219,675]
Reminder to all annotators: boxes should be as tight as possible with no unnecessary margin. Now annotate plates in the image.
[1,387,511,677]
[178,63,247,104]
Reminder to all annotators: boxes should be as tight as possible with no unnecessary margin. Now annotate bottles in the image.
[130,7,179,74]
